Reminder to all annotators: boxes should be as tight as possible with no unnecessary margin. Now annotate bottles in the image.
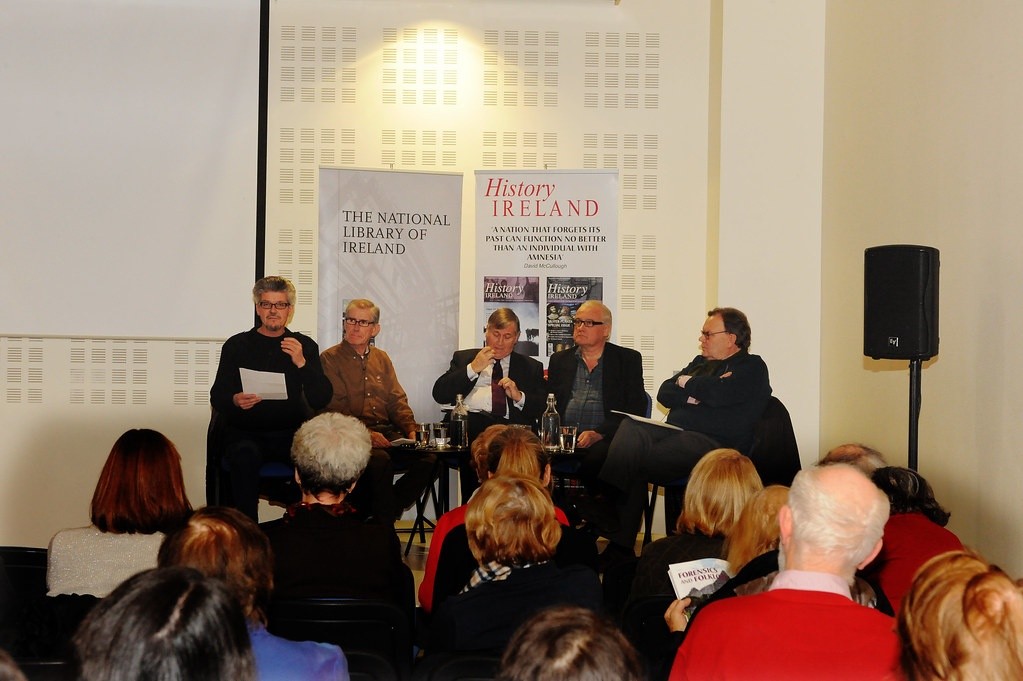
[449,394,467,448]
[542,394,561,450]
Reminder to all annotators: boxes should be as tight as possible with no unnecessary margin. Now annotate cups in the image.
[559,427,577,453]
[415,422,431,447]
[433,422,450,450]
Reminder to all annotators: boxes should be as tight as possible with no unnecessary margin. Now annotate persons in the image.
[579,309,772,575]
[664,463,911,681]
[432,307,546,507]
[470,424,511,501]
[210,276,334,504]
[417,426,569,612]
[258,411,403,599]
[547,299,648,535]
[301,299,443,522]
[46,428,195,599]
[631,448,764,593]
[495,605,645,681]
[867,466,966,616]
[64,565,255,681]
[412,474,604,661]
[158,506,351,681]
[725,485,791,571]
[821,443,886,480]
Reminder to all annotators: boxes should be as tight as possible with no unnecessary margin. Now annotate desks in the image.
[396,442,613,562]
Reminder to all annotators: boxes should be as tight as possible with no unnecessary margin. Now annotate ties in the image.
[491,358,506,416]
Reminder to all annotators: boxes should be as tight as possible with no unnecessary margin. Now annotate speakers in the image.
[863,245,940,361]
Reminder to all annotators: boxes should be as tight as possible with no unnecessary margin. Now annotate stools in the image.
[262,462,440,555]
[640,468,689,552]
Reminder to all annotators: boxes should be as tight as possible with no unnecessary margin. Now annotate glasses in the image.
[573,318,604,326]
[701,330,729,340]
[259,302,289,309]
[345,317,376,327]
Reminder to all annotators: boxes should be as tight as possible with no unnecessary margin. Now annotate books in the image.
[610,409,684,431]
[667,558,735,627]
